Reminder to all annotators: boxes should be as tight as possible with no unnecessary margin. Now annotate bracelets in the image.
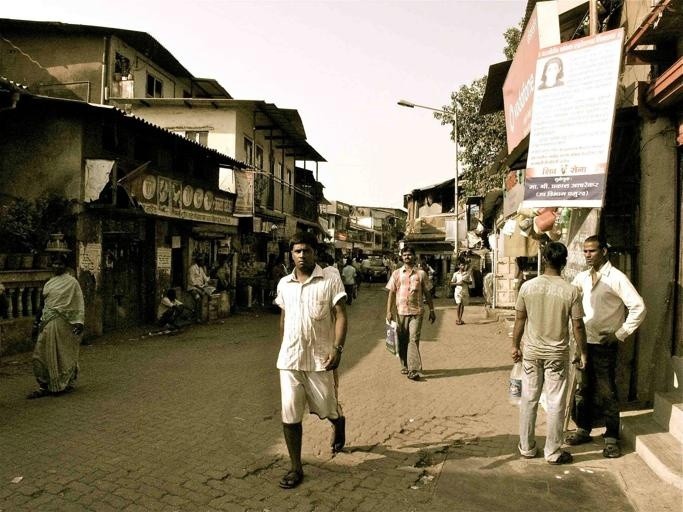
[429,308,435,311]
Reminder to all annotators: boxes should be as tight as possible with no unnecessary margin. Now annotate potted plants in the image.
[0,188,78,272]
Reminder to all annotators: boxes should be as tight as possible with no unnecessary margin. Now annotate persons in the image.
[563,234,648,459]
[25,253,86,400]
[419,195,443,218]
[150,249,292,330]
[384,246,437,380]
[448,259,473,327]
[319,251,440,305]
[273,231,350,489]
[537,57,565,91]
[509,241,588,464]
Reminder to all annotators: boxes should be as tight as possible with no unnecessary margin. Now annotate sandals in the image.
[401,369,408,374]
[408,371,421,380]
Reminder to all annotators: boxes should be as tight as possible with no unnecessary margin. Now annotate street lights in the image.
[395,99,459,258]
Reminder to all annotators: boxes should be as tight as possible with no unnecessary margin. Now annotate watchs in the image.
[332,343,345,353]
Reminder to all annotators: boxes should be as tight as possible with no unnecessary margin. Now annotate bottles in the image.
[508,359,522,406]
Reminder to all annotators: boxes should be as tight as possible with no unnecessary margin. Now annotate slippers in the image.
[604,443,619,458]
[279,470,301,489]
[564,432,592,444]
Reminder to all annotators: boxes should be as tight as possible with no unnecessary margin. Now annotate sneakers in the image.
[550,452,572,465]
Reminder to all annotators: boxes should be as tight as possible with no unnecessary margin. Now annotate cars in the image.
[361,259,388,282]
